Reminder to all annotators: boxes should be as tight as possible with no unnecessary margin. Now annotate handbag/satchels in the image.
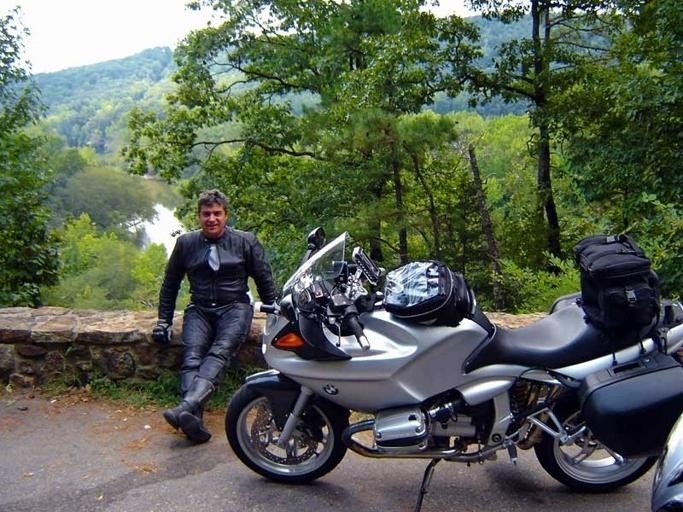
[571,233,663,334]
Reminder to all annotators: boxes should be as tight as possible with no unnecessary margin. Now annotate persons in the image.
[150,187,278,444]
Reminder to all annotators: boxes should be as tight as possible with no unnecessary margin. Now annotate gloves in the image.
[151,319,173,344]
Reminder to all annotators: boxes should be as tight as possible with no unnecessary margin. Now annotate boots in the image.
[163,378,216,430]
[177,405,211,443]
